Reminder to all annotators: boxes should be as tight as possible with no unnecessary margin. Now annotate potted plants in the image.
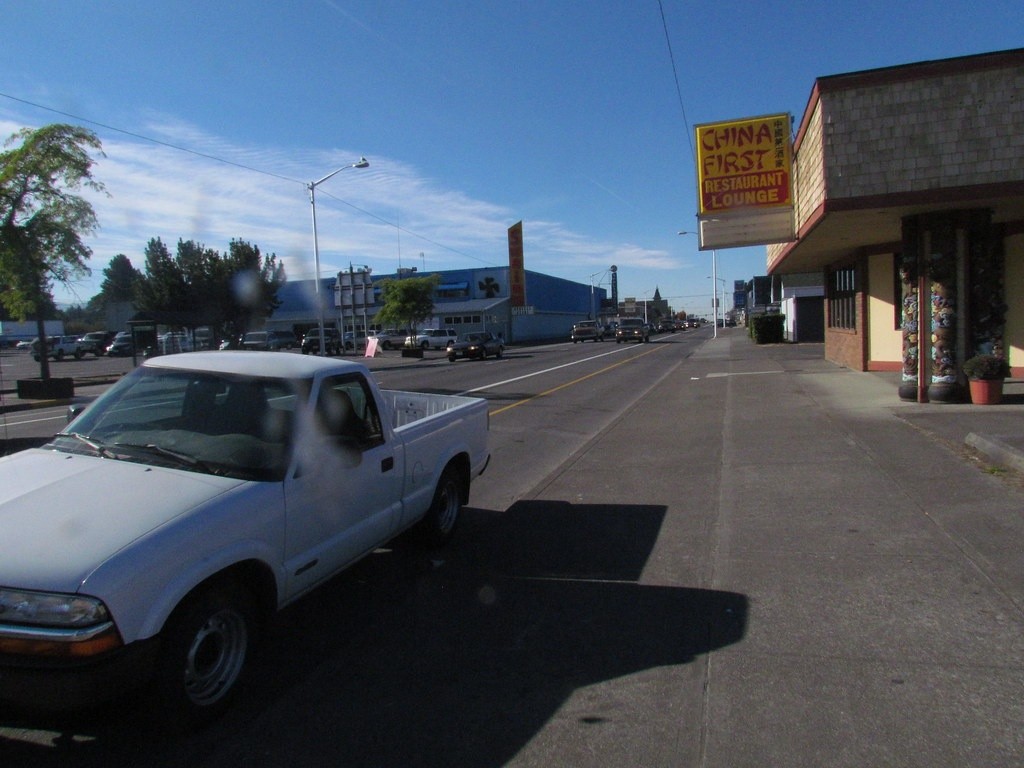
[962,353,1011,405]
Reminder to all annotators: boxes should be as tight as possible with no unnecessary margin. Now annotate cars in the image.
[77,329,303,358]
[17,339,33,351]
[571,319,605,344]
[446,330,505,363]
[605,322,619,337]
[343,328,380,350]
[646,318,699,334]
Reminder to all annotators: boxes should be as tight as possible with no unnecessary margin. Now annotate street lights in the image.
[706,276,728,329]
[308,159,370,356]
[677,231,718,339]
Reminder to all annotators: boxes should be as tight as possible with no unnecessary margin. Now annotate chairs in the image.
[205,388,266,437]
[314,391,365,446]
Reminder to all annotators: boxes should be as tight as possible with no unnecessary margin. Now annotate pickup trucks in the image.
[0,348,493,735]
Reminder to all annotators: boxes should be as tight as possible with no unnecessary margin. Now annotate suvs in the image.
[375,328,416,350]
[30,335,85,360]
[615,318,651,344]
[404,328,457,350]
[300,328,343,354]
